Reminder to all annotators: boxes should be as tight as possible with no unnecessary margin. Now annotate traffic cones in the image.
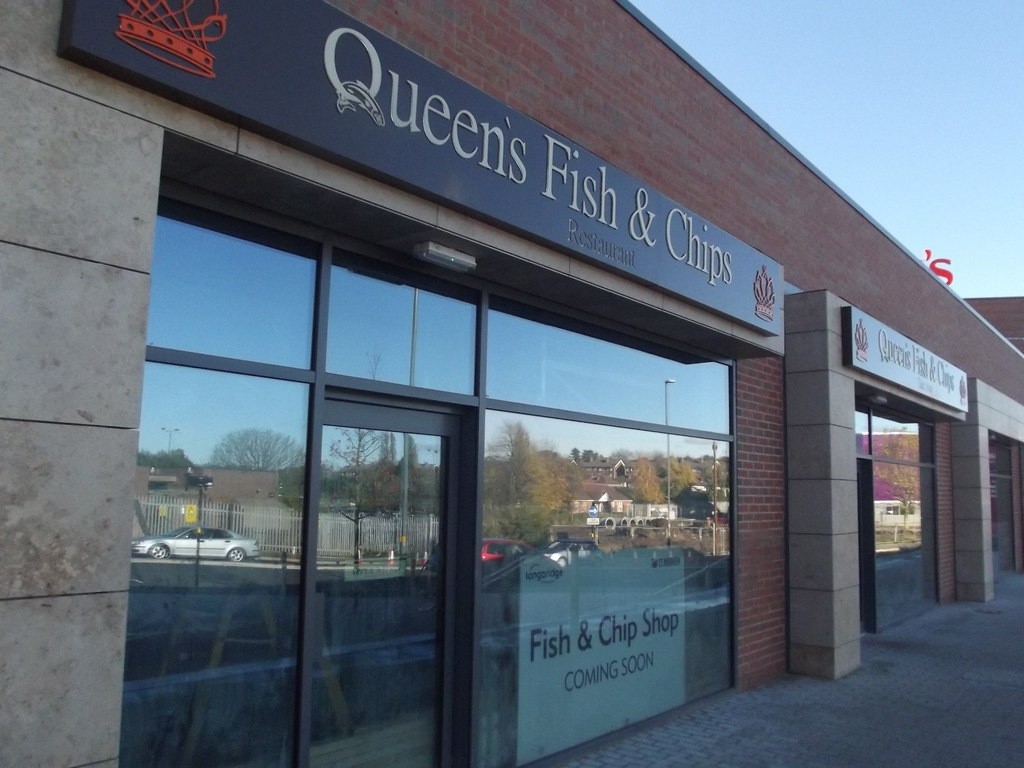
[358,549,362,564]
[388,548,395,566]
[422,549,428,566]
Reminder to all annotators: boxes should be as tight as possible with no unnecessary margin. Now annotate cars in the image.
[481,538,534,560]
[540,540,602,568]
[130,527,260,562]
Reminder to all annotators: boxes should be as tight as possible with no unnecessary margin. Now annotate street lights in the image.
[162,428,180,453]
[665,380,676,536]
